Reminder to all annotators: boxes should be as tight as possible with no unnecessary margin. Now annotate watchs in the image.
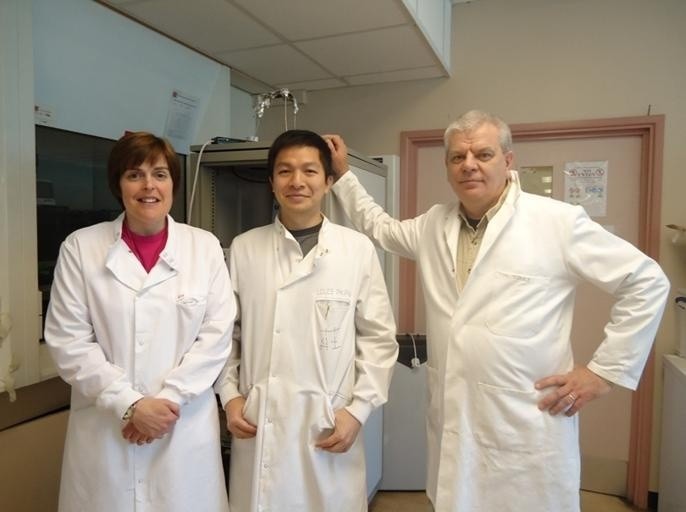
[123,397,143,421]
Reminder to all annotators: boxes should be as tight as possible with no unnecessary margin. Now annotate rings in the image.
[568,393,575,401]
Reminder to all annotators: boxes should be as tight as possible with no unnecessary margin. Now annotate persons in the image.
[212,128,402,512]
[322,109,673,510]
[42,131,237,511]
